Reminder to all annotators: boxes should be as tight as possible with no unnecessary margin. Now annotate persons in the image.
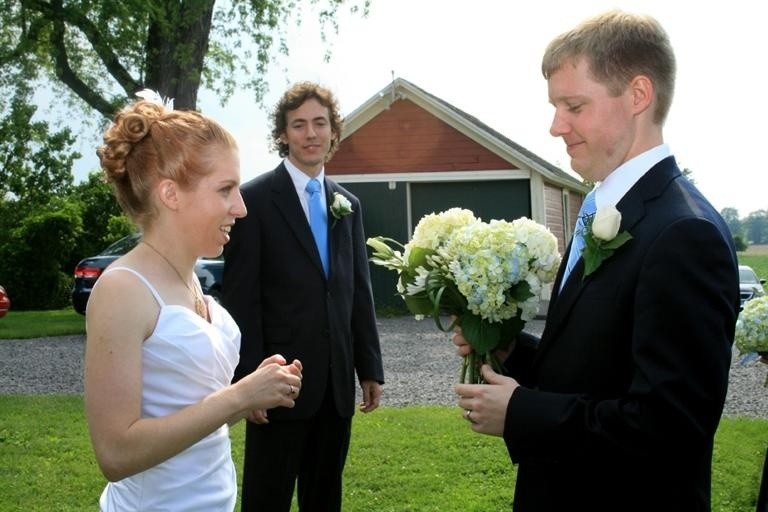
[221,80,386,512]
[83,90,304,512]
[450,6,740,511]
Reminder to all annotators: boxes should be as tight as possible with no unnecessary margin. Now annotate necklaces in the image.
[139,240,208,320]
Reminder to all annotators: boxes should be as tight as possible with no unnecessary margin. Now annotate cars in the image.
[0,285,11,318]
[737,264,767,311]
[71,230,226,316]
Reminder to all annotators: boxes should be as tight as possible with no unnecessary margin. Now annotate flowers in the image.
[359,200,572,388]
[326,189,357,232]
[729,293,768,389]
[574,204,635,285]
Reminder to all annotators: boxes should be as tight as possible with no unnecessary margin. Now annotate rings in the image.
[466,409,472,422]
[287,385,297,397]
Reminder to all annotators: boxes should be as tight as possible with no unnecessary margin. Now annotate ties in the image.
[304,177,338,281]
[556,186,601,303]
